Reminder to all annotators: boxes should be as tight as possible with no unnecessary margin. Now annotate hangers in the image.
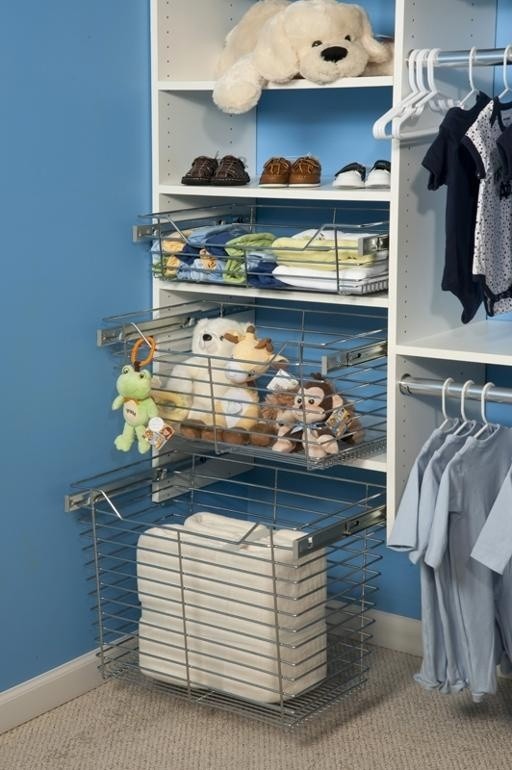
[437,377,498,438]
[373,45,512,140]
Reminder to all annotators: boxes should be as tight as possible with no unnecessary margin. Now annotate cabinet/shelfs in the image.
[64,0,512,733]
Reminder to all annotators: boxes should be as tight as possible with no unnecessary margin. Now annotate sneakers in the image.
[210,155,250,185]
[364,160,391,188]
[289,157,322,187]
[333,162,366,188]
[259,158,292,187]
[181,156,217,185]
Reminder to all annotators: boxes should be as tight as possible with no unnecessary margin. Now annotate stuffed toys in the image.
[172,317,253,438]
[249,379,364,444]
[203,331,287,444]
[112,364,158,455]
[211,0,391,115]
[273,373,339,459]
